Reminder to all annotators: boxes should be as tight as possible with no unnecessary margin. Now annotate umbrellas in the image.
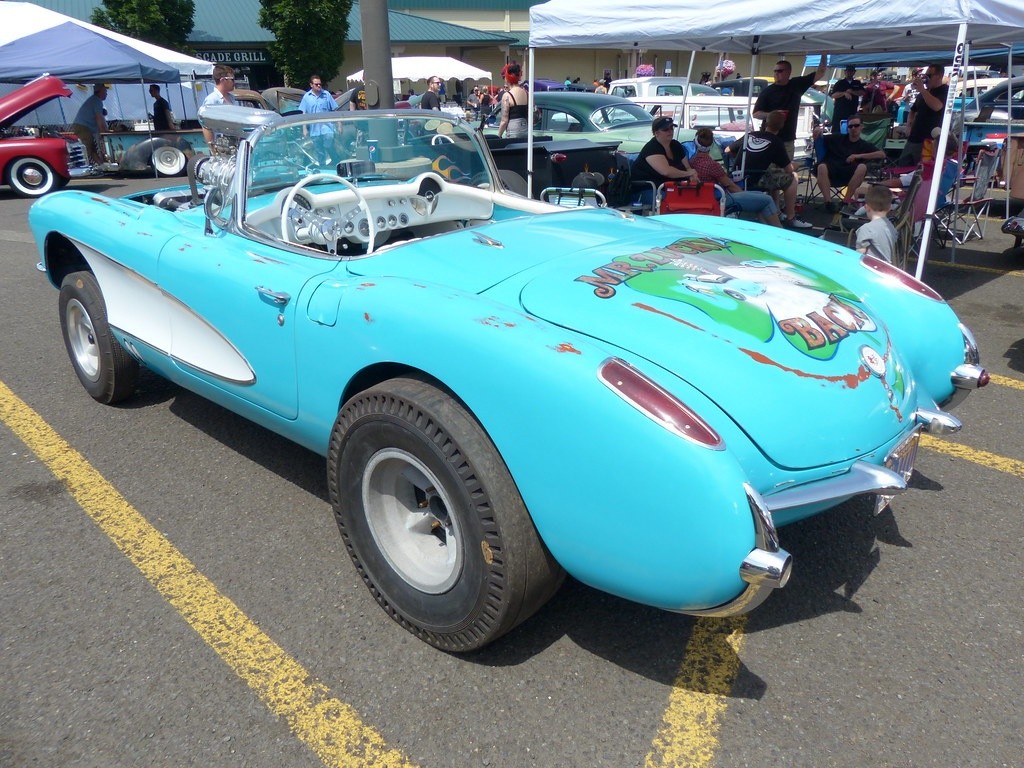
[346,56,491,88]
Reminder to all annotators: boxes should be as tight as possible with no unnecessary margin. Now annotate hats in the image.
[844,66,856,72]
[599,79,606,84]
[94,82,109,89]
[652,116,678,132]
[912,67,924,76]
[571,172,605,188]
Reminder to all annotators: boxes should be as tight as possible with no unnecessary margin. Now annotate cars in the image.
[0,71,90,198]
[445,92,740,180]
[540,68,1024,165]
[25,114,995,658]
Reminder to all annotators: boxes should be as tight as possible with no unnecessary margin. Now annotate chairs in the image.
[540,188,606,209]
[615,113,1001,270]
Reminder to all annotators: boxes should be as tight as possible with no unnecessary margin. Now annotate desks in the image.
[100,129,210,164]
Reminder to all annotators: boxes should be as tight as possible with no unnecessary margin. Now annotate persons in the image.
[593,72,613,94]
[421,76,442,111]
[932,133,960,209]
[408,88,420,110]
[348,70,370,148]
[467,85,496,128]
[148,83,176,131]
[688,128,785,229]
[199,64,240,155]
[573,77,580,84]
[300,75,344,166]
[72,83,109,166]
[492,61,530,136]
[725,109,813,228]
[629,115,699,204]
[897,64,950,169]
[564,77,571,84]
[735,72,741,79]
[854,185,898,264]
[812,116,887,215]
[833,64,927,134]
[752,53,827,168]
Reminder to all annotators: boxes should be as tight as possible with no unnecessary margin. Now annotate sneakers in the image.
[824,202,834,213]
[784,215,813,228]
[839,198,859,211]
[779,213,787,222]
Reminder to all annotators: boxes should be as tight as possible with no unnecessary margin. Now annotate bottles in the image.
[608,168,615,194]
[571,163,599,191]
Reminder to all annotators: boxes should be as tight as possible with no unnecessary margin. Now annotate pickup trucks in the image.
[96,86,304,178]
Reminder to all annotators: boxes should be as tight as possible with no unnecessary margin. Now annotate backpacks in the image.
[607,165,632,207]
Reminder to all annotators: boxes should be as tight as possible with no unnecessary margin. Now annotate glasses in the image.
[313,83,322,86]
[431,82,441,86]
[503,86,509,89]
[222,76,235,83]
[926,73,938,79]
[848,124,862,129]
[774,69,788,73]
[149,89,157,92]
[660,126,674,132]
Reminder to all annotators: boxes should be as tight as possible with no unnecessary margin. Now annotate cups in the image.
[911,76,929,90]
[732,170,742,182]
[367,139,378,163]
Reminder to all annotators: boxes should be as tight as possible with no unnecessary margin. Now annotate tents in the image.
[525,0,1024,280]
[0,1,234,127]
[1,20,187,179]
[828,43,1024,116]
